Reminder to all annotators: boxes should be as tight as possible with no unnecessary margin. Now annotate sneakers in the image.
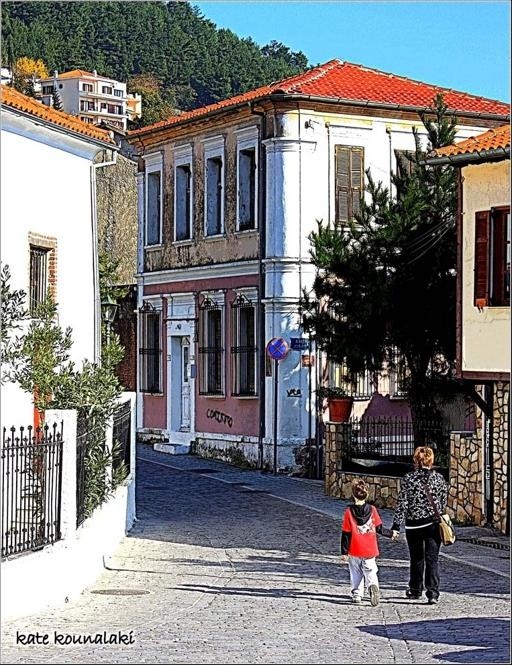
[405,588,422,600]
[428,597,439,605]
[369,585,379,607]
[351,596,361,604]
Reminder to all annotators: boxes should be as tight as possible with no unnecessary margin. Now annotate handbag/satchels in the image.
[436,514,457,547]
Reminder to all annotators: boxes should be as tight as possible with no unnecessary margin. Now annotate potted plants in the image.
[318,385,356,422]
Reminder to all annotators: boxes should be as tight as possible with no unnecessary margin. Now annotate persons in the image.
[340,479,399,608]
[389,445,449,605]
